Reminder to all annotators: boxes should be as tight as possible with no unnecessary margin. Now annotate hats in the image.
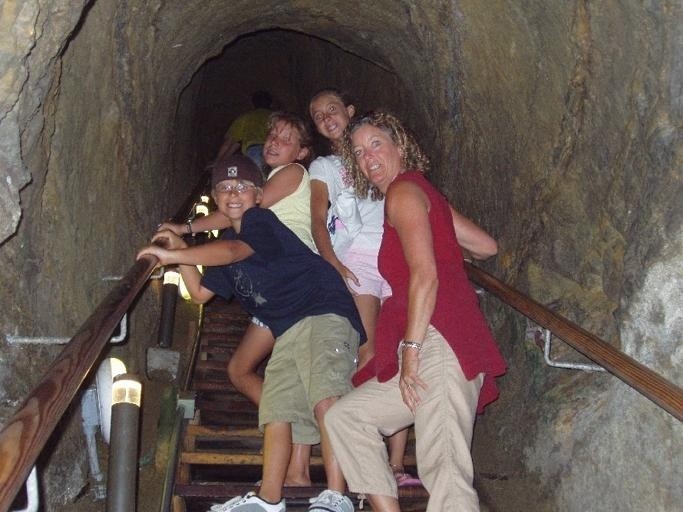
[207,151,264,186]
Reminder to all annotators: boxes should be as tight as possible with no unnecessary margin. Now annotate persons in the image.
[137,153,367,512]
[325,106,499,512]
[157,109,319,487]
[308,90,423,488]
[215,87,287,176]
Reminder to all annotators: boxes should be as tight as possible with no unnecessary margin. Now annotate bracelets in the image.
[401,341,421,349]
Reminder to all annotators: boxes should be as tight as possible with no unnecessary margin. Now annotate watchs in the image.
[185,220,194,236]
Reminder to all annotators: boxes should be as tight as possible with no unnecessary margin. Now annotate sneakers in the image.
[393,472,427,487]
[208,490,288,512]
[305,486,357,512]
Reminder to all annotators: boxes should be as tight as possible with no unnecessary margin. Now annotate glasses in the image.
[213,184,258,196]
